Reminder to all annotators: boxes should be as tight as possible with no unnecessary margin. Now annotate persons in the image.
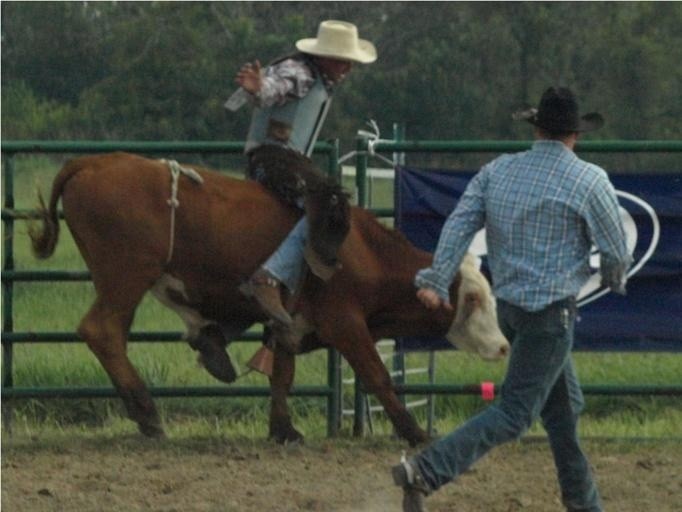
[185,19,381,381]
[388,83,634,510]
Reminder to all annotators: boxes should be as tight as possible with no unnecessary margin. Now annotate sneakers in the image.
[183,329,239,386]
[389,456,426,511]
[235,266,294,325]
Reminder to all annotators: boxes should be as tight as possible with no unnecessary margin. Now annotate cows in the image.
[25,150,511,449]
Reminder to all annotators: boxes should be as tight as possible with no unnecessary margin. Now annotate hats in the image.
[510,84,607,136]
[293,17,379,67]
